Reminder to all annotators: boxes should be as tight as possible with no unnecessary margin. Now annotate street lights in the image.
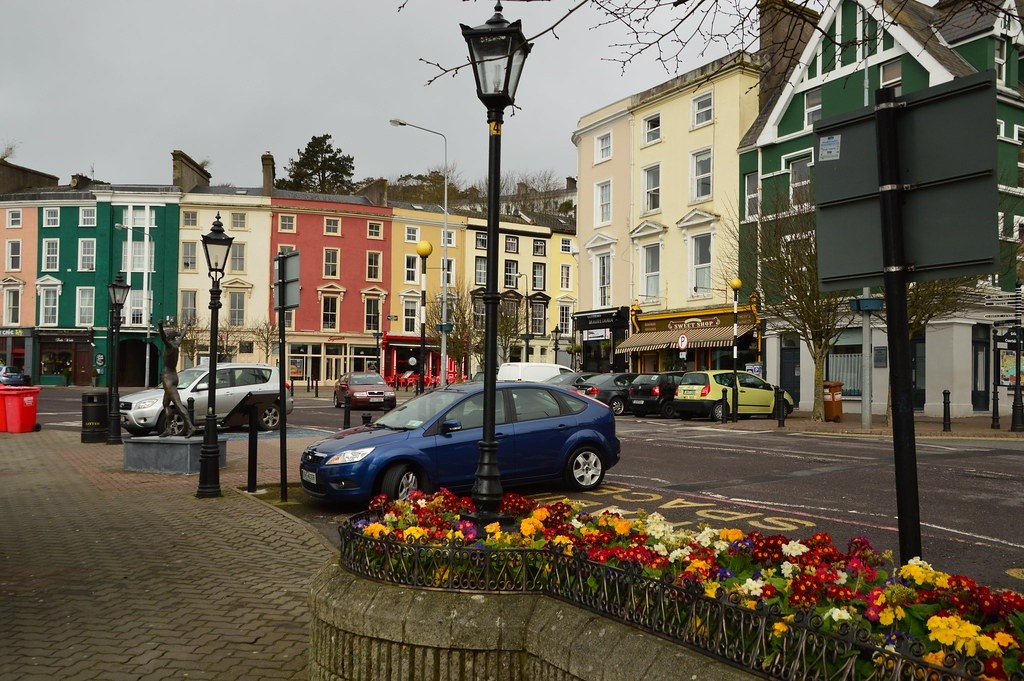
[196,212,236,498]
[105,271,131,445]
[416,242,433,392]
[115,223,152,388]
[514,272,530,362]
[729,278,742,422]
[391,119,448,388]
[551,325,562,364]
[460,0,534,541]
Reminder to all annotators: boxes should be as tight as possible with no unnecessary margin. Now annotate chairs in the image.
[464,410,485,429]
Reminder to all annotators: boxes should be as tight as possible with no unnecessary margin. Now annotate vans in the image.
[498,361,585,386]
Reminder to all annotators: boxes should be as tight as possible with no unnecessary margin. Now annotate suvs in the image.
[627,371,690,419]
[119,362,294,437]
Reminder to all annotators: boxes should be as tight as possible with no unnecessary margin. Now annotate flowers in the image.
[355,485,1024,681]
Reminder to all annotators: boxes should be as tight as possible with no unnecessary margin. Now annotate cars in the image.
[472,371,497,382]
[0,366,25,386]
[672,369,793,420]
[541,371,639,416]
[301,381,620,513]
[334,372,396,410]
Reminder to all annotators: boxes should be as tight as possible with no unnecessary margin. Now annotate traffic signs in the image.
[984,294,1022,325]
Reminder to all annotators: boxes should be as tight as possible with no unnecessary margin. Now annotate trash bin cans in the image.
[81,392,109,442]
[823,380,845,423]
[0,384,11,432]
[4,386,41,433]
